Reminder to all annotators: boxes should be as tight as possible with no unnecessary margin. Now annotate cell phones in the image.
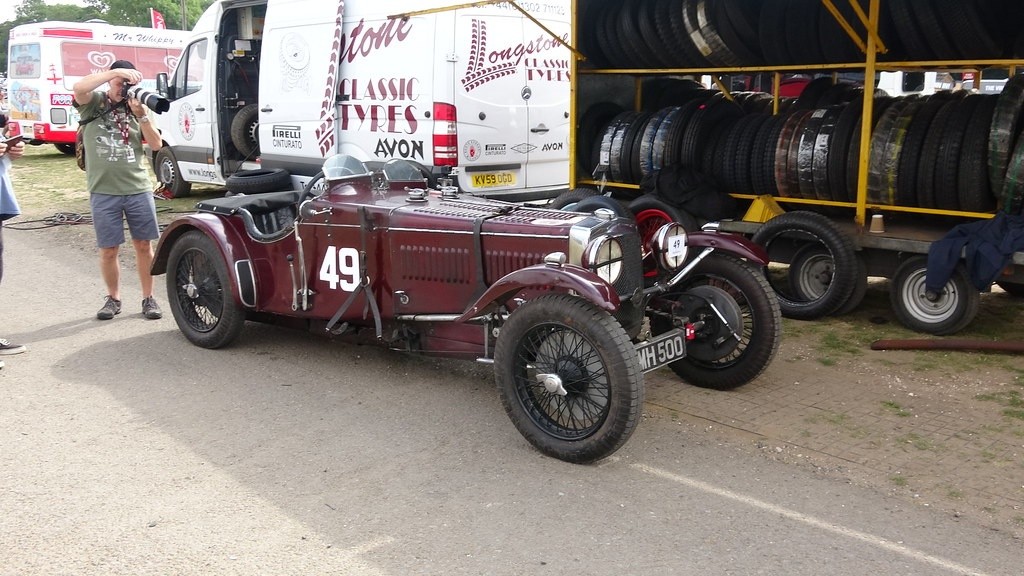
[1,135,23,152]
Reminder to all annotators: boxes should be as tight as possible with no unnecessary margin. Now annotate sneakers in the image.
[142,297,162,319]
[97,295,122,320]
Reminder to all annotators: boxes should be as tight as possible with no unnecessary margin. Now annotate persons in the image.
[0,84,25,354]
[72,60,163,319]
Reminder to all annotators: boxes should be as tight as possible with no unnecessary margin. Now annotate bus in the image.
[7,21,190,154]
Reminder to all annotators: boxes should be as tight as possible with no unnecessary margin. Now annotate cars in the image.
[147,155,784,466]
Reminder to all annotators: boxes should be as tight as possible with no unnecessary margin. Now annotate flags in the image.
[150,7,166,29]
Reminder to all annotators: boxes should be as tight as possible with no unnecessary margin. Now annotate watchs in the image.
[134,115,149,123]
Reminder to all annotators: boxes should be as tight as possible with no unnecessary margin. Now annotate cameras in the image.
[122,78,170,115]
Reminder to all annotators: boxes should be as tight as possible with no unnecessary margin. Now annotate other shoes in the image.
[0,338,26,355]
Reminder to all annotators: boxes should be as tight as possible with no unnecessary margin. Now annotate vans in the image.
[141,0,571,203]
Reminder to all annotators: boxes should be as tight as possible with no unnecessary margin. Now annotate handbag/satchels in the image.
[75,121,86,172]
